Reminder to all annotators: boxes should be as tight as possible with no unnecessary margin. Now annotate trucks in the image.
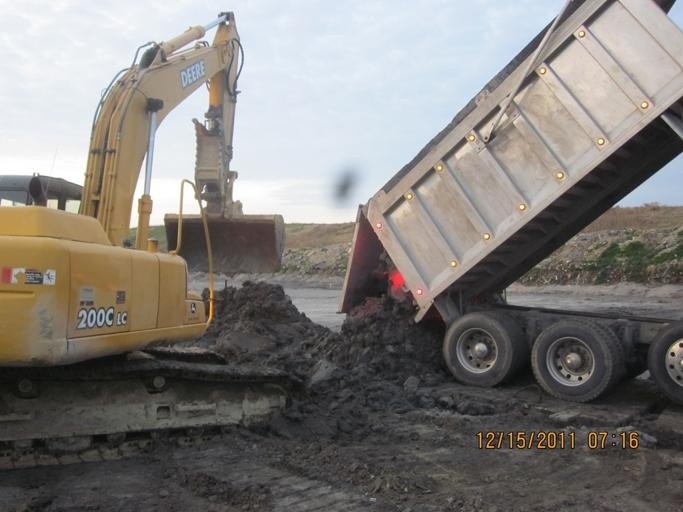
[340,0,683,411]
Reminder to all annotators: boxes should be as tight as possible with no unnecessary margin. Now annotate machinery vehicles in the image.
[1,11,286,396]
